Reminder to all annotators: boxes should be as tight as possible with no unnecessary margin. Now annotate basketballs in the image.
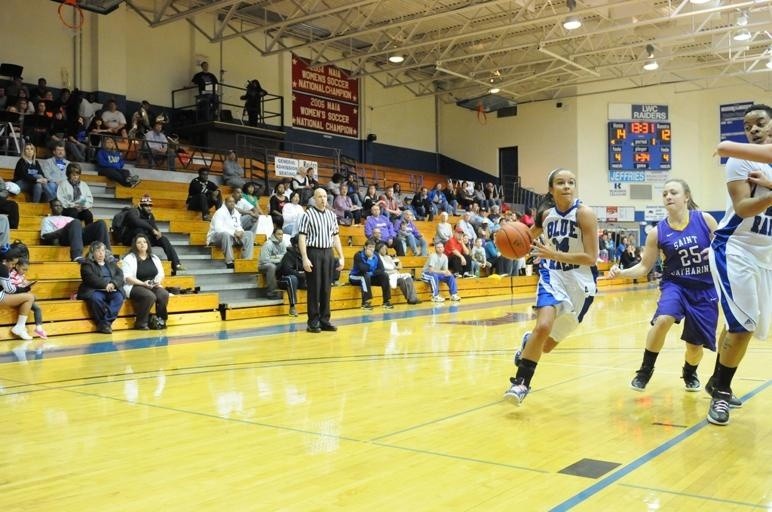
[496,221,534,259]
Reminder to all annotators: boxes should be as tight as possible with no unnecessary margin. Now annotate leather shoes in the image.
[226,263,234,269]
[176,264,187,271]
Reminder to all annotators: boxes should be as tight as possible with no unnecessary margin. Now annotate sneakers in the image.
[504,376,531,406]
[514,331,533,366]
[704,378,745,406]
[11,326,33,342]
[266,271,477,333]
[34,328,48,341]
[96,324,113,334]
[630,364,654,392]
[139,326,150,330]
[681,366,702,393]
[707,396,731,427]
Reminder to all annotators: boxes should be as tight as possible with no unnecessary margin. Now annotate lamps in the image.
[486,79,501,96]
[387,35,406,64]
[562,1,585,31]
[640,44,659,71]
[733,16,751,43]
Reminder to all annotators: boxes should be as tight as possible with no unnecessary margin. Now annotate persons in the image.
[241,80,268,128]
[323,170,428,255]
[347,228,422,310]
[1,143,181,340]
[598,228,662,280]
[711,118,772,191]
[410,180,504,218]
[434,203,545,278]
[299,188,346,333]
[1,76,176,188]
[256,167,340,317]
[187,61,220,121]
[704,104,772,426]
[184,149,264,269]
[501,167,601,407]
[606,177,719,393]
[421,242,463,301]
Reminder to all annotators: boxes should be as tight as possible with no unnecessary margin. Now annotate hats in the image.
[140,194,153,205]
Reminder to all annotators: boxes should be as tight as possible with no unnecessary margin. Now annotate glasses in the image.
[53,204,63,208]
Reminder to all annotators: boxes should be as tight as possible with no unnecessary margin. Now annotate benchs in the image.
[181,145,638,314]
[0,88,181,344]
